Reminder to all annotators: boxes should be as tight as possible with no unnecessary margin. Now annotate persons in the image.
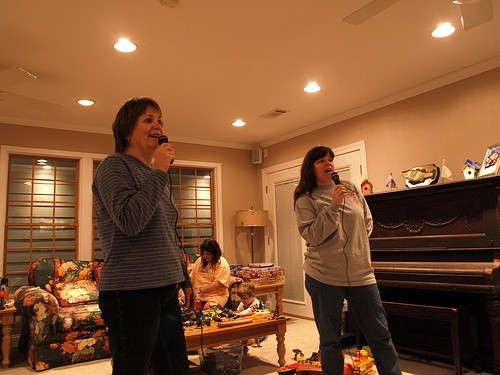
[236,282,264,344]
[294,146,401,375]
[188,239,230,311]
[92,96,196,375]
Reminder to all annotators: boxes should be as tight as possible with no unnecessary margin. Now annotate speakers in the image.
[251,148,261,164]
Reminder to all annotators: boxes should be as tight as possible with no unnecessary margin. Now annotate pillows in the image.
[52,279,100,307]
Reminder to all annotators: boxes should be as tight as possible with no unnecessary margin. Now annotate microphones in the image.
[331,172,341,186]
[158,135,174,163]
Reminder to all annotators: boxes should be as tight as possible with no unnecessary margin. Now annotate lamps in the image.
[235,206,268,264]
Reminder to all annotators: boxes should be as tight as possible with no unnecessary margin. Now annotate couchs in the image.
[13,256,243,372]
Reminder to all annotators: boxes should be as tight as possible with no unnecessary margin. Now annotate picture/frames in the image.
[477,142,500,178]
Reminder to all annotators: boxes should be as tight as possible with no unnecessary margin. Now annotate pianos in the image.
[335,174,500,375]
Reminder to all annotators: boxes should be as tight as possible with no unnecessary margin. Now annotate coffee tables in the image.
[183,309,291,375]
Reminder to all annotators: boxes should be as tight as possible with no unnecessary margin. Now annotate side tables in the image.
[229,264,285,315]
[0,299,17,370]
